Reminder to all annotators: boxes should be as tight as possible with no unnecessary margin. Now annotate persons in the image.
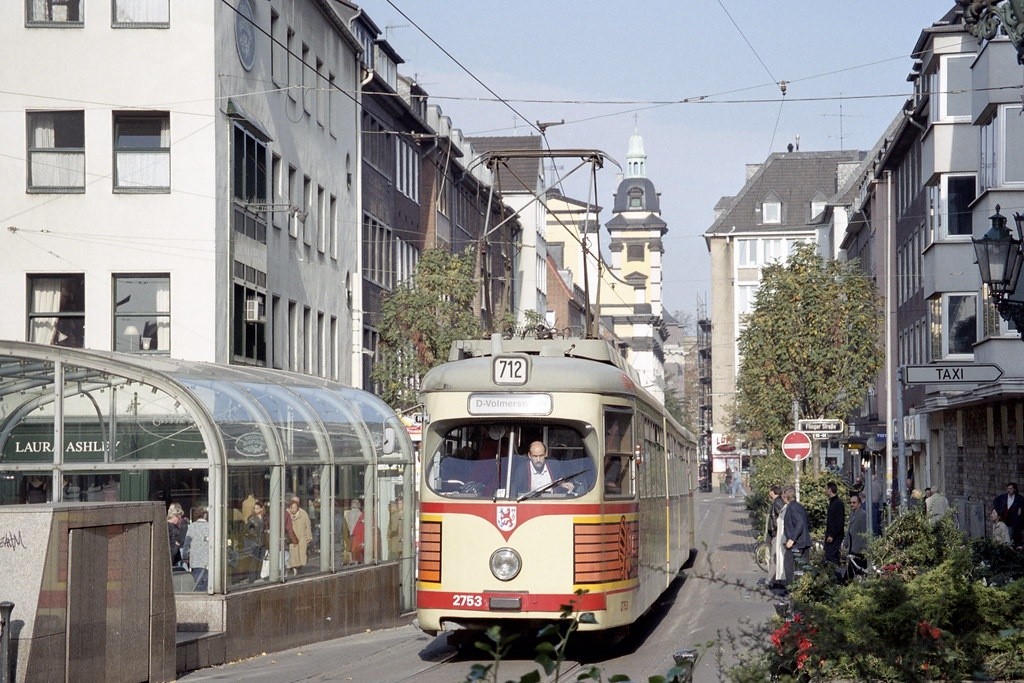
[824,481,846,565]
[990,482,1024,550]
[842,494,867,587]
[512,441,575,497]
[905,467,948,528]
[725,463,749,498]
[766,483,814,616]
[167,485,404,592]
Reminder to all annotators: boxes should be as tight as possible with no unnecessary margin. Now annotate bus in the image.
[382,333,699,631]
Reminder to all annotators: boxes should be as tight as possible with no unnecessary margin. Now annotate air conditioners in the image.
[245,299,263,321]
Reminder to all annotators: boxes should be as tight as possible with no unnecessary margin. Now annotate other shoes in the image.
[742,494,747,500]
[729,495,735,498]
[758,583,769,590]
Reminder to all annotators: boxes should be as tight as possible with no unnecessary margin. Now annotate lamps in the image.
[243,200,310,223]
[972,201,1024,339]
[123,324,139,352]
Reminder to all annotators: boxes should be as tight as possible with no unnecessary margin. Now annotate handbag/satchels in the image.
[260,549,269,579]
[284,533,293,545]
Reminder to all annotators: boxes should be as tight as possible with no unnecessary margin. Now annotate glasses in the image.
[850,502,856,504]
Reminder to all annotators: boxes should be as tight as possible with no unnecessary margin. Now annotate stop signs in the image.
[782,431,812,461]
